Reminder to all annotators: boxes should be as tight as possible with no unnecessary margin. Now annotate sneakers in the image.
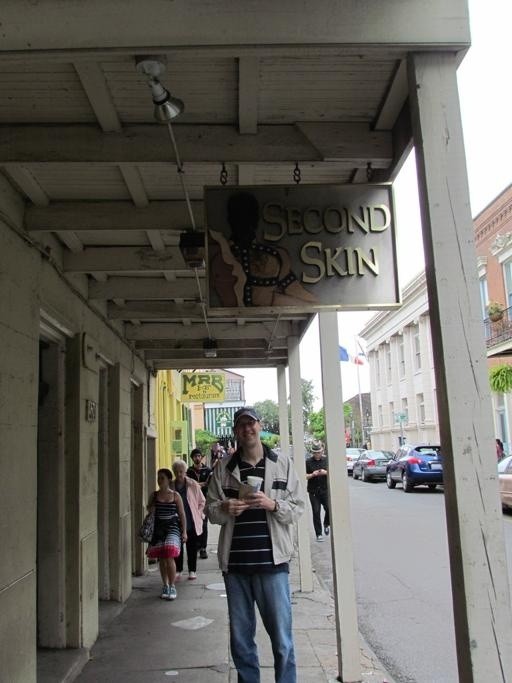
[200,549,207,558]
[324,524,329,535]
[316,533,322,541]
[169,585,177,599]
[189,570,196,578]
[175,570,181,580]
[161,584,168,597]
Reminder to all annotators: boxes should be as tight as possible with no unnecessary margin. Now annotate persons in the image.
[496,438,504,463]
[145,468,188,600]
[204,407,305,683]
[306,438,330,542]
[171,460,207,580]
[209,440,236,471]
[186,448,213,560]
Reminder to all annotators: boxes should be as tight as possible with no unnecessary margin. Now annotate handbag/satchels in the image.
[138,490,156,542]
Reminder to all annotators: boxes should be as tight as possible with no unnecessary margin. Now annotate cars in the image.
[346,447,366,477]
[497,454,512,514]
[352,449,395,482]
[385,443,444,492]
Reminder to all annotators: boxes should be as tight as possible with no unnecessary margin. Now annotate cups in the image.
[248,476,262,492]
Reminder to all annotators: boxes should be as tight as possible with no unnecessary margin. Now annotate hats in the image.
[190,448,201,455]
[234,407,260,427]
[309,439,325,453]
